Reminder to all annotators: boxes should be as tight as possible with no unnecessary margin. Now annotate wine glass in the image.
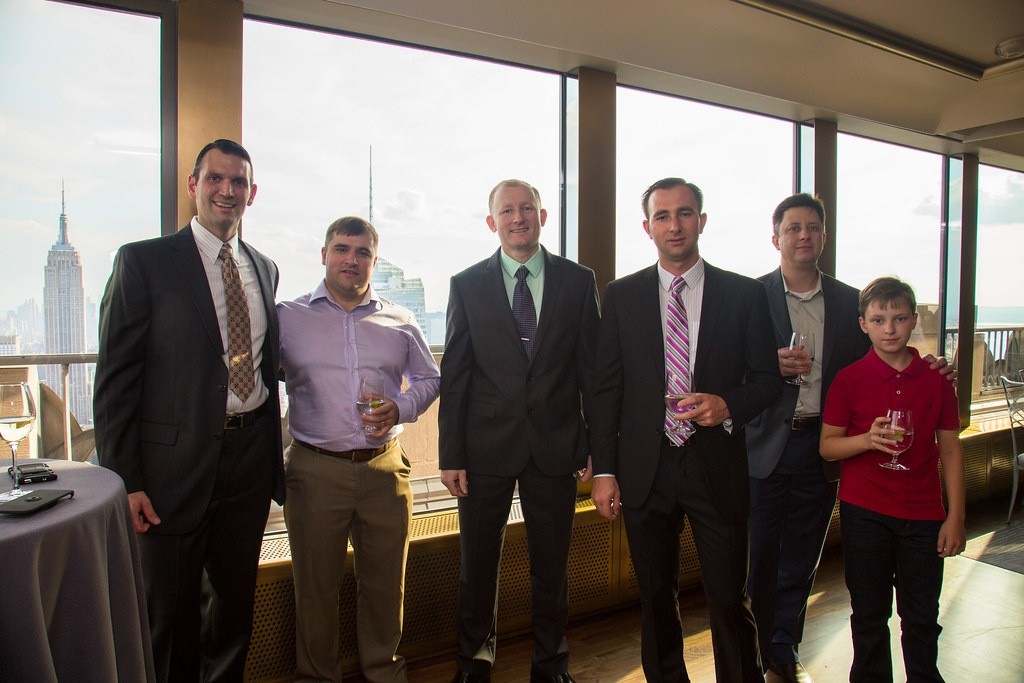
[0,381,37,502]
[355,375,385,434]
[879,408,913,471]
[785,330,815,386]
[666,370,697,434]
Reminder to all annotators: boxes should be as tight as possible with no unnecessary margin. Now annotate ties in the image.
[218,243,255,403]
[511,266,537,363]
[663,277,697,449]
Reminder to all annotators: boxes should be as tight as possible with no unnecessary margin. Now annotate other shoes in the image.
[757,649,814,683]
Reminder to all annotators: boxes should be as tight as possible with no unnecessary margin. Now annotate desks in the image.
[0,456,158,683]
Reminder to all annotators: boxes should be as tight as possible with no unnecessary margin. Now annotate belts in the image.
[663,432,690,450]
[292,438,397,465]
[791,416,821,432]
[224,406,265,431]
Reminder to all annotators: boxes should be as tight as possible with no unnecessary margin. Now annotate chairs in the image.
[1000,376,1024,524]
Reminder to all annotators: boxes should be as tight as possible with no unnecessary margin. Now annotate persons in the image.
[819,277,969,683]
[93,139,288,682]
[439,180,601,682]
[746,193,958,683]
[587,177,764,683]
[274,215,439,682]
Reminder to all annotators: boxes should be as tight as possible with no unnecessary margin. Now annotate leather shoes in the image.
[530,670,576,683]
[451,668,491,683]
